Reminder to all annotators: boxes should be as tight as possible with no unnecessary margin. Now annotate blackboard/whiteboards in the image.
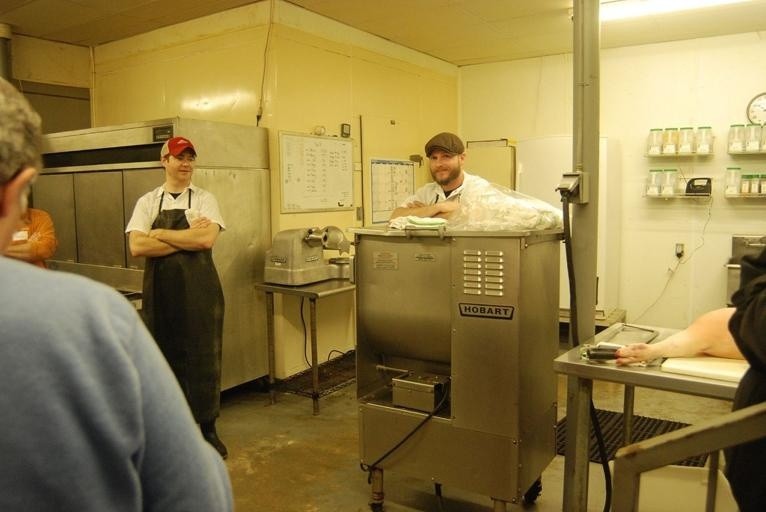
[278,129,356,215]
[458,147,516,194]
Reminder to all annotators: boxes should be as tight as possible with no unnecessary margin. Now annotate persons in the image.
[391,130,488,221]
[2,204,58,271]
[121,133,230,462]
[1,74,235,510]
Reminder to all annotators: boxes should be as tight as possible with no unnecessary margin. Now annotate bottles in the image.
[727,122,766,151]
[724,167,766,194]
[646,126,713,154]
[646,168,680,196]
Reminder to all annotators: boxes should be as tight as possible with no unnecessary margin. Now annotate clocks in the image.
[746,92,765,125]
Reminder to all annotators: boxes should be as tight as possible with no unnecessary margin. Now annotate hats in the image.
[425,132,464,156]
[160,137,197,158]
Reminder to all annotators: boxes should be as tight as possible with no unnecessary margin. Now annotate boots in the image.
[201,419,228,461]
